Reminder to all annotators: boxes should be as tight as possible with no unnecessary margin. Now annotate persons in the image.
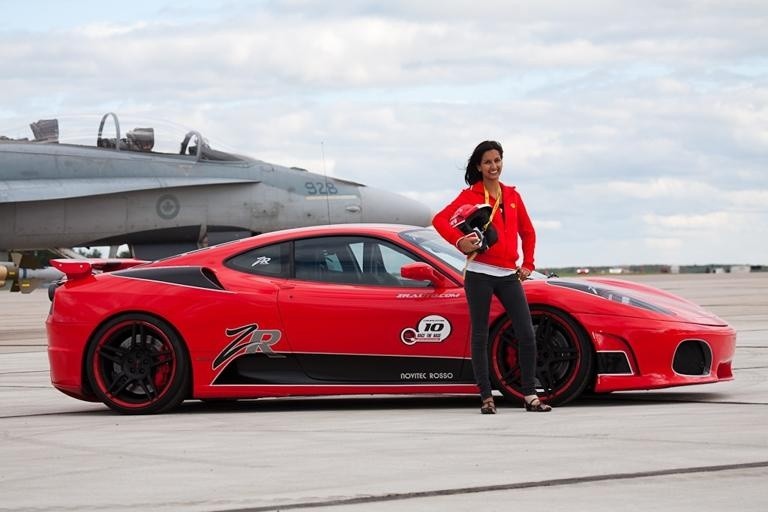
[432,141,553,414]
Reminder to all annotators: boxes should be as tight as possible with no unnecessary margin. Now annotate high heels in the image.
[524,396,551,412]
[481,397,497,414]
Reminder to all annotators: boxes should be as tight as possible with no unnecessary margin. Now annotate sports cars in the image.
[47,222,736,417]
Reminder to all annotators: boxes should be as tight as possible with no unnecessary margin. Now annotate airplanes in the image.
[1,111,438,299]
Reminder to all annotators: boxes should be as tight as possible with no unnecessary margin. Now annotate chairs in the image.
[363,242,403,286]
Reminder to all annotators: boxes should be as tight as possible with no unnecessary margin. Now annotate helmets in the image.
[449,203,498,255]
